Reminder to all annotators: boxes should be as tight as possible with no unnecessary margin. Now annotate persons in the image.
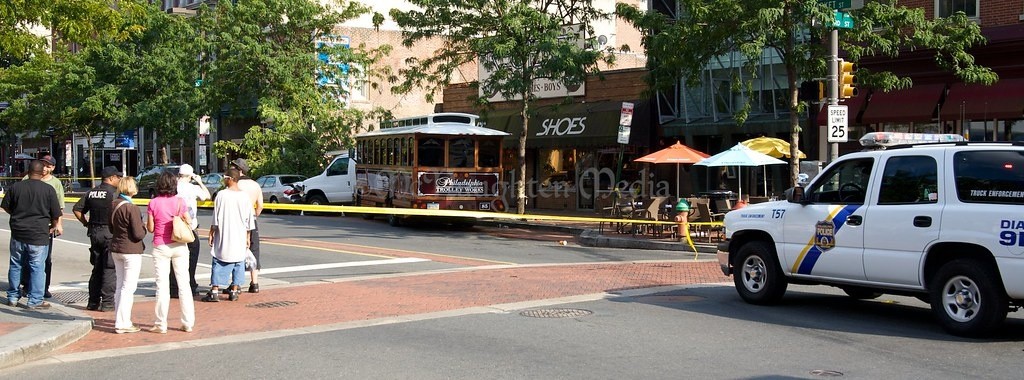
[111,176,146,335]
[147,170,197,334]
[0,159,265,309]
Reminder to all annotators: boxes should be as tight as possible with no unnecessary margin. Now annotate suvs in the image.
[281,153,358,216]
[714,131,1024,340]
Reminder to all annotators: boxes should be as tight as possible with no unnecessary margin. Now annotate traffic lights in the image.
[839,61,860,99]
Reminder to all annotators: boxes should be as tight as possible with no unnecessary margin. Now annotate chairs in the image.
[597,197,732,244]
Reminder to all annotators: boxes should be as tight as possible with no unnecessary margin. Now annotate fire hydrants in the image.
[673,198,692,243]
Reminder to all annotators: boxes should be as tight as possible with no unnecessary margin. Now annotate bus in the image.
[351,110,514,232]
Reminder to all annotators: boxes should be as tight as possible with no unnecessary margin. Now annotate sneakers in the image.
[248,281,259,292]
[229,290,238,300]
[201,290,220,302]
[223,284,241,294]
[114,324,141,334]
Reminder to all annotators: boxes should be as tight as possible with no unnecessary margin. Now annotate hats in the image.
[178,164,195,176]
[40,155,56,166]
[101,166,123,178]
[231,158,248,175]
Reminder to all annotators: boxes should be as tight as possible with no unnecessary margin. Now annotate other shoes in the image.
[86,303,103,310]
[22,290,28,297]
[27,300,50,310]
[148,325,168,333]
[101,305,115,312]
[179,326,192,332]
[44,291,51,298]
[8,301,18,307]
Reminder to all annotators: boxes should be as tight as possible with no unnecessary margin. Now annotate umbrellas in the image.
[634,136,806,204]
[10,153,36,171]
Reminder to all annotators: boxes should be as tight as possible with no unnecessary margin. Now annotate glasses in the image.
[223,174,233,179]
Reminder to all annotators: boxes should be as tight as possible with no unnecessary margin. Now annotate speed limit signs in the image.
[827,104,850,144]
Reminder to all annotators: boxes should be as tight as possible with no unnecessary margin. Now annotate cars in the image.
[254,176,309,215]
[191,172,228,201]
[134,161,182,198]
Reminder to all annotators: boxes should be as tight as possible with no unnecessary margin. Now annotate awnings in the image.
[818,78,1024,124]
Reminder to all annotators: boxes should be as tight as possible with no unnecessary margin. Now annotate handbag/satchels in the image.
[171,198,195,243]
[244,247,257,271]
